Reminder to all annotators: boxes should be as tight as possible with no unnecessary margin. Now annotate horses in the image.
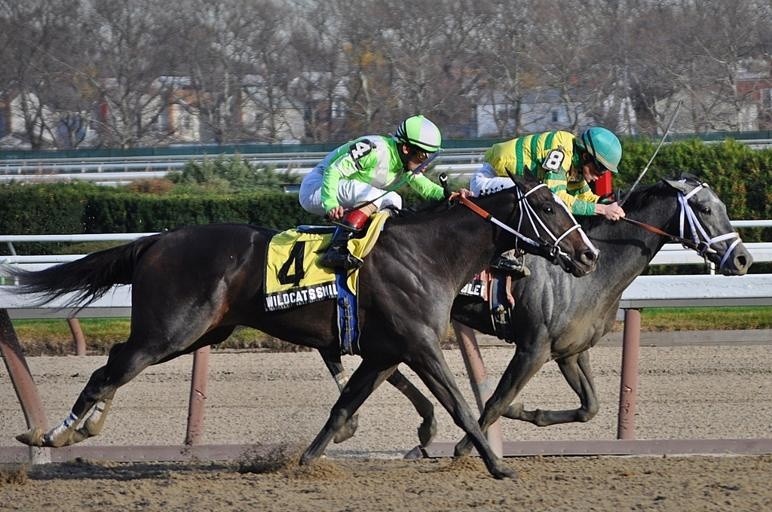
[316,164,754,462]
[0,163,602,483]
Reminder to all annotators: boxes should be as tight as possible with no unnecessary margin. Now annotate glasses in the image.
[416,149,429,163]
[595,159,607,172]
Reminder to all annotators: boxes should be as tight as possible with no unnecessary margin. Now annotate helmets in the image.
[393,114,441,152]
[576,127,622,174]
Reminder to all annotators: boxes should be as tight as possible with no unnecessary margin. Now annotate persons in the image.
[471,273,482,295]
[471,127,626,278]
[298,114,474,270]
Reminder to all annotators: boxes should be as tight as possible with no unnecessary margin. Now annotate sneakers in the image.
[491,255,521,270]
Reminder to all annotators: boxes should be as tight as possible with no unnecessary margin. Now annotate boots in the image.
[320,210,368,266]
